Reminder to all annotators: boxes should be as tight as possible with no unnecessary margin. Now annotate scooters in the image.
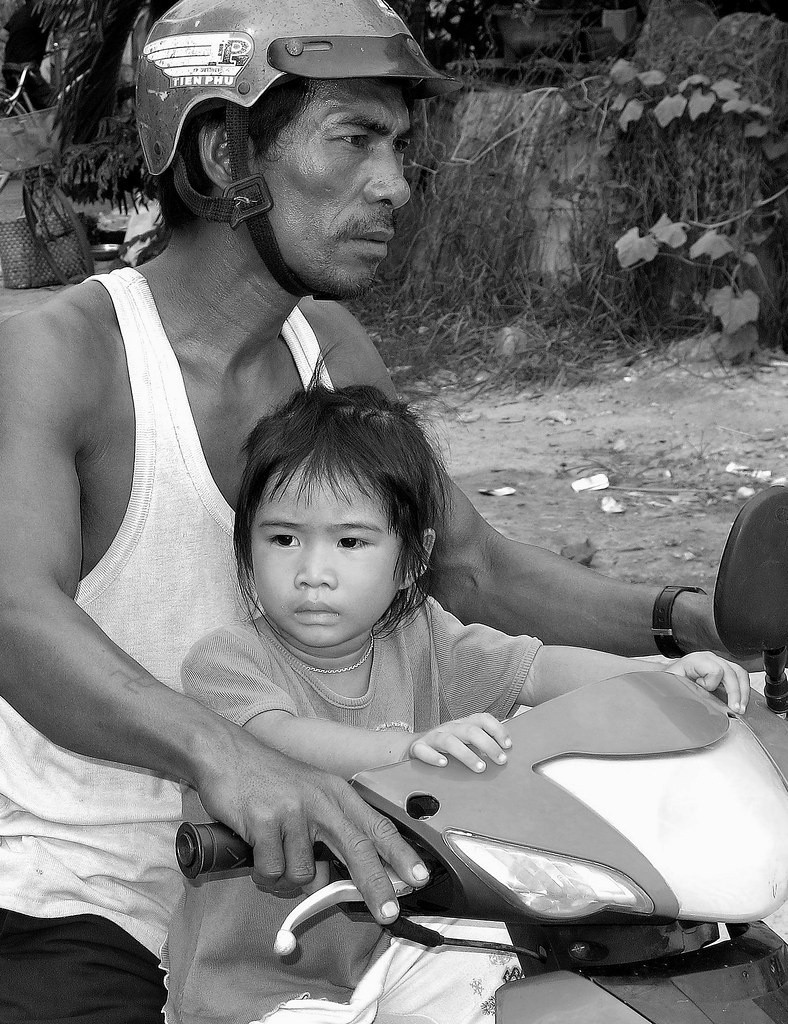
[180,488,788,1024]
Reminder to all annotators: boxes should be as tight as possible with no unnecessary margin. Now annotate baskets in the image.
[0,106,62,171]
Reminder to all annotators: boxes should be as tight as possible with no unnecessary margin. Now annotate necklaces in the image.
[297,633,373,674]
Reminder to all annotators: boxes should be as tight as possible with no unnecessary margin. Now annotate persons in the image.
[0,2,767,1023]
[163,386,751,1024]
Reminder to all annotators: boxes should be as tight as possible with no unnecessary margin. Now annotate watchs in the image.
[651,585,707,660]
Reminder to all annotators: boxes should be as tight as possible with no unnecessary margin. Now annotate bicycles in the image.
[0,60,98,285]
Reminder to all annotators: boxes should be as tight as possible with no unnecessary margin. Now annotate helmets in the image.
[135,0,459,176]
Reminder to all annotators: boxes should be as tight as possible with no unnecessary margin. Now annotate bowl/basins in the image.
[89,243,121,261]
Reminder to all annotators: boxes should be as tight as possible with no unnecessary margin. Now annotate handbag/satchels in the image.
[0,202,88,289]
